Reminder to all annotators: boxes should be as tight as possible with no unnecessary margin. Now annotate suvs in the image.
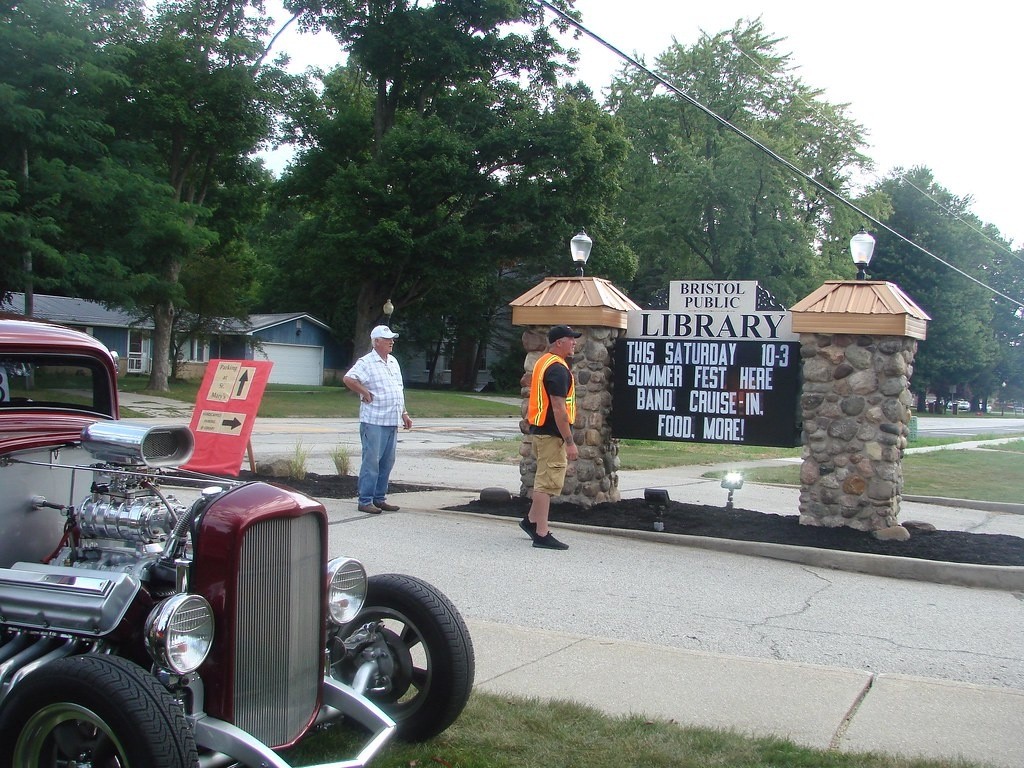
[0,311,475,768]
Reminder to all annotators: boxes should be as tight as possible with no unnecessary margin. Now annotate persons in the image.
[343,325,413,514]
[519,325,582,550]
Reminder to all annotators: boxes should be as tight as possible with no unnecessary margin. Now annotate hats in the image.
[370,325,399,338]
[547,325,582,344]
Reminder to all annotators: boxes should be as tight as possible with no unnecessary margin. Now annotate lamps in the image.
[570,225,593,277]
[721,474,744,508]
[643,488,670,531]
[849,224,876,281]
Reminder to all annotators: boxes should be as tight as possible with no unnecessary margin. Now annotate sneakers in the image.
[358,503,382,513]
[374,501,400,511]
[519,516,537,539]
[533,531,569,550]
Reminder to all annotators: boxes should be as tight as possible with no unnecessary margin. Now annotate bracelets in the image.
[403,411,407,414]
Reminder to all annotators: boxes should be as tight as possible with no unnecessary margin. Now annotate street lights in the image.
[383,299,394,327]
[1002,381,1006,416]
